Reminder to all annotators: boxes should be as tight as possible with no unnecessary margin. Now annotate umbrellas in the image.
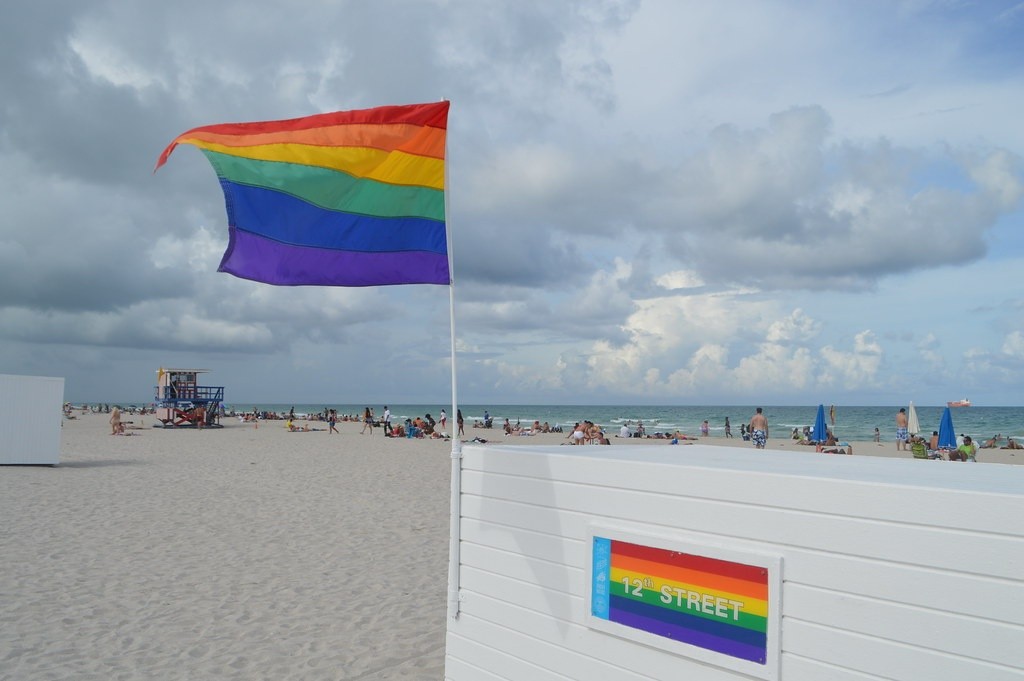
[216,404,229,409]
[812,405,828,453]
[906,401,921,442]
[937,408,957,460]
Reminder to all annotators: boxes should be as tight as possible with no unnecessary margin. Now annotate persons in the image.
[360,406,436,438]
[64,402,71,412]
[503,419,550,436]
[564,420,608,445]
[287,406,359,434]
[620,422,699,440]
[109,406,125,435]
[457,409,493,435]
[724,417,733,438]
[130,407,155,415]
[440,409,446,429]
[741,407,1024,462]
[241,409,277,422]
[702,419,709,436]
[220,408,225,416]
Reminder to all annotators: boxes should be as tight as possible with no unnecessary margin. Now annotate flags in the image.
[152,99,452,290]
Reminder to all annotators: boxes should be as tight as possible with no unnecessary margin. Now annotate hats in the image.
[425,414,430,417]
[406,419,411,421]
